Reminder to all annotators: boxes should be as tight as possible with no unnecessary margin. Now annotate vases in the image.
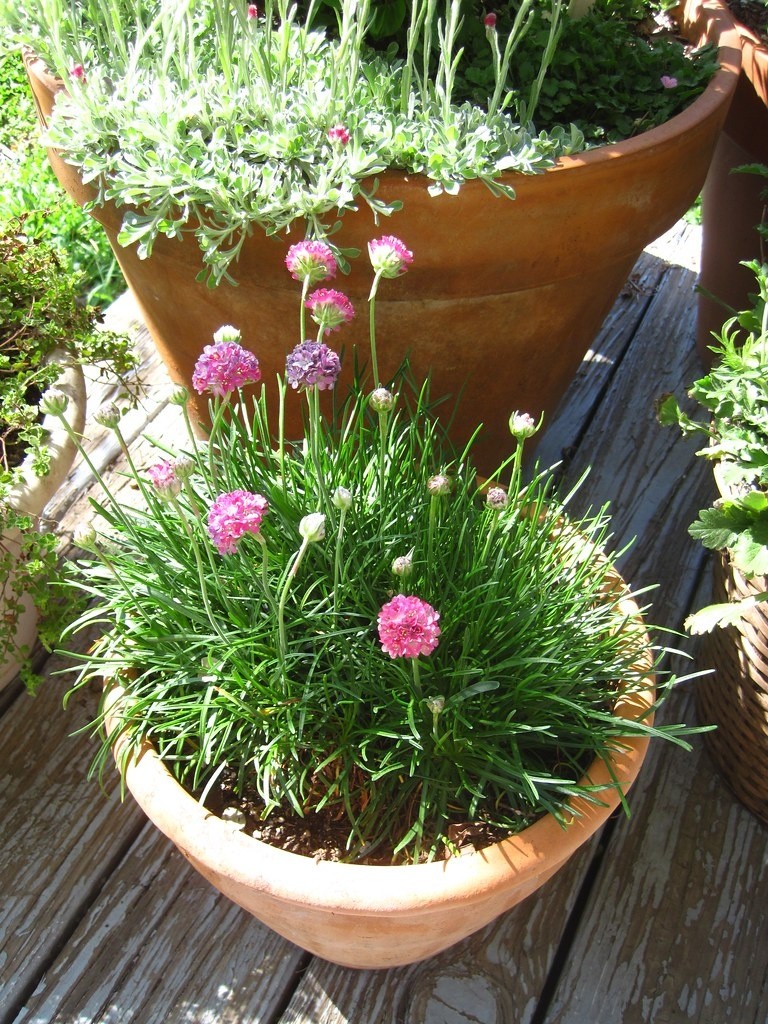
[96,469,659,971]
[33,0,761,497]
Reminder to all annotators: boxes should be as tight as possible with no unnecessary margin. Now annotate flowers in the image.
[5,0,723,275]
[48,235,721,867]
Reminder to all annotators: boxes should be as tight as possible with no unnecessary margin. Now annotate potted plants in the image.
[0,231,150,696]
